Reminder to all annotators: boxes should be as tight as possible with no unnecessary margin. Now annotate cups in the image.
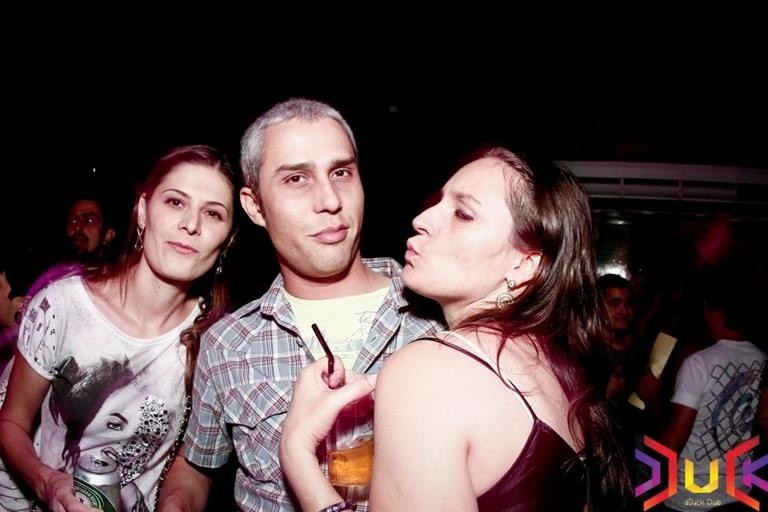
[322,396,375,507]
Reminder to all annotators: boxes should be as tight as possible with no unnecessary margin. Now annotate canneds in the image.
[73,450,123,511]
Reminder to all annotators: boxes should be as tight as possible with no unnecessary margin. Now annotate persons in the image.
[2,101,768,512]
